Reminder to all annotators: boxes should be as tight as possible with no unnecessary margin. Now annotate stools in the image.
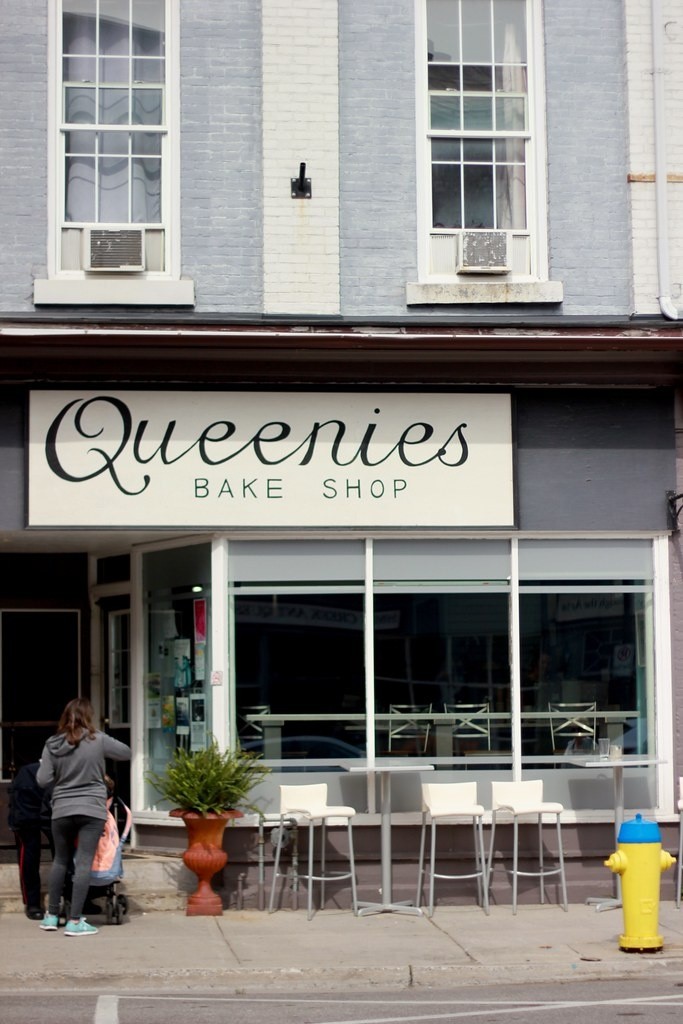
[483,779,568,915]
[416,781,490,916]
[268,783,358,921]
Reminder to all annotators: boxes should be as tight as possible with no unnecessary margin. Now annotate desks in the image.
[248,711,641,720]
[339,761,434,918]
[570,756,668,912]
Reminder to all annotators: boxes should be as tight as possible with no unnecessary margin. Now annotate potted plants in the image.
[143,729,268,917]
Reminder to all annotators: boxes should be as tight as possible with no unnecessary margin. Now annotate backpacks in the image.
[73,795,133,885]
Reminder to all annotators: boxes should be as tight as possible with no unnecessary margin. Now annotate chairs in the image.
[547,701,597,770]
[376,704,433,757]
[443,703,512,771]
[237,706,271,740]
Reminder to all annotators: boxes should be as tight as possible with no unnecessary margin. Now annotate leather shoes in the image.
[25,906,44,920]
[82,901,102,914]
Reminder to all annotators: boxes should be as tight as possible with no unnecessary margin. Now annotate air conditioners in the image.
[82,226,146,272]
[455,229,513,275]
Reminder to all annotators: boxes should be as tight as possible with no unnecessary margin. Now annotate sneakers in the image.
[39,916,58,931]
[63,918,99,936]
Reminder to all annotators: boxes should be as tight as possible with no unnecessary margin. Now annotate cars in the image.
[239,738,436,774]
[566,716,653,774]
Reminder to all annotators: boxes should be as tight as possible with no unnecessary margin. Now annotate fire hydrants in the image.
[603,812,676,950]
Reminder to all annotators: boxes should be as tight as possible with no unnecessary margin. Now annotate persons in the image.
[7,699,131,937]
[522,647,554,708]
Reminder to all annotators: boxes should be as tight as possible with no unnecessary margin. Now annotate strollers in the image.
[61,787,131,924]
